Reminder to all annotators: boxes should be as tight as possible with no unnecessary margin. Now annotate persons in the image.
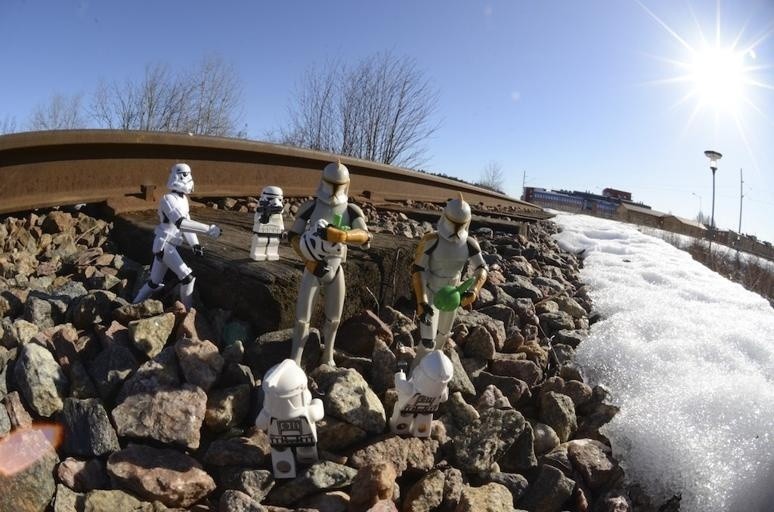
[385,349,455,439]
[245,184,289,262]
[285,155,375,371]
[133,159,224,310]
[254,358,326,479]
[410,191,489,378]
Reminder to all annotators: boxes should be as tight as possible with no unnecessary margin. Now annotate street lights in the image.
[704,150,722,226]
